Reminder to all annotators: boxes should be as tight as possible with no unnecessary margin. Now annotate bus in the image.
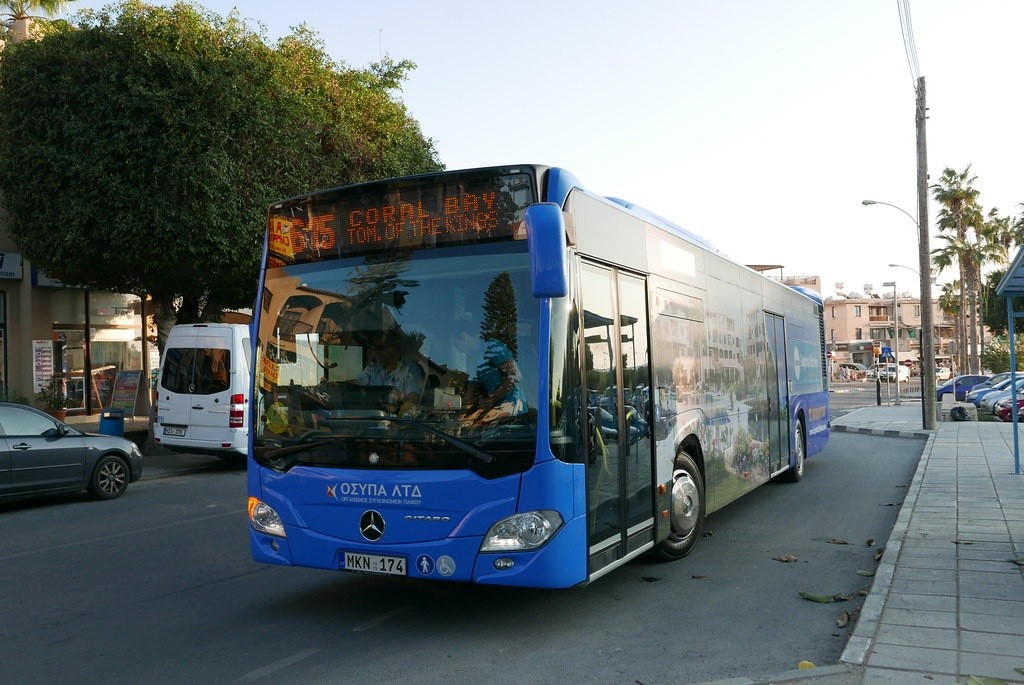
[246,163,831,599]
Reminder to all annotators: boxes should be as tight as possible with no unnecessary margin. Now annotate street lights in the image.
[861,198,941,432]
[882,282,904,406]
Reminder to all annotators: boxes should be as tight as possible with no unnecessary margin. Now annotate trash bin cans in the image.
[98,406,125,437]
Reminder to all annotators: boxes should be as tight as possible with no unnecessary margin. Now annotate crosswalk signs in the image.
[881,347,892,358]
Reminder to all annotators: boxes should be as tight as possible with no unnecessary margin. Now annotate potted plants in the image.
[40,375,75,422]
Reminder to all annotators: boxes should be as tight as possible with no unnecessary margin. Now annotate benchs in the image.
[941,394,979,421]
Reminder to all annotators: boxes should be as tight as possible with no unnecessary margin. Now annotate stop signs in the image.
[905,359,912,368]
[827,352,832,358]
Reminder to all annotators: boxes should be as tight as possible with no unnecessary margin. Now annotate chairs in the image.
[398,325,433,404]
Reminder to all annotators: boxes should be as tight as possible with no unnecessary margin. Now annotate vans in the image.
[154,324,365,466]
[880,365,911,383]
[869,363,896,380]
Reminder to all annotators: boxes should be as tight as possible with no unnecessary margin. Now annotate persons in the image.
[449,320,529,416]
[320,330,427,406]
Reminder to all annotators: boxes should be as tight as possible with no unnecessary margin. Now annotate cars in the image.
[935,367,951,381]
[981,379,1024,417]
[587,385,652,444]
[971,372,1024,393]
[936,375,997,403]
[994,388,1024,422]
[0,402,144,505]
[840,363,874,378]
[966,376,1024,407]
